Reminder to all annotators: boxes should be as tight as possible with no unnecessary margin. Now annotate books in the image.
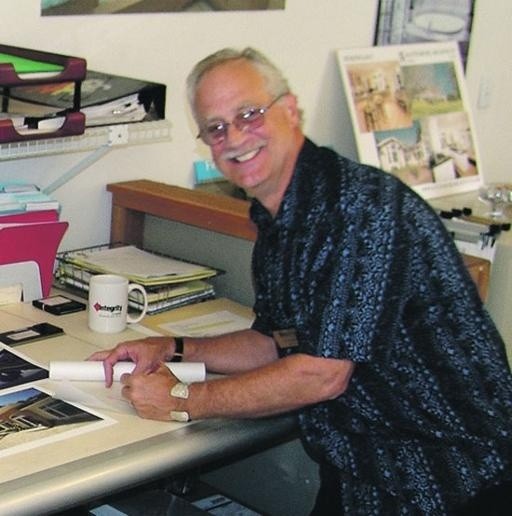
[52,240,226,316]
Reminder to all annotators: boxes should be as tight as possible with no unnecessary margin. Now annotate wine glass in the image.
[476,182,512,222]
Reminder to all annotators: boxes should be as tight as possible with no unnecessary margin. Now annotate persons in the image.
[85,47,511,516]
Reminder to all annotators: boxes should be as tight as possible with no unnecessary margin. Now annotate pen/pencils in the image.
[144,360,163,376]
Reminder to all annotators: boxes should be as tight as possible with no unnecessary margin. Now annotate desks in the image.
[449,245,491,311]
[0,268,331,516]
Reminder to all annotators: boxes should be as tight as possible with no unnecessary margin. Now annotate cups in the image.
[88,274,148,335]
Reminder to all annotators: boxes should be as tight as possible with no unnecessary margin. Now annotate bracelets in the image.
[169,379,193,424]
[170,334,184,365]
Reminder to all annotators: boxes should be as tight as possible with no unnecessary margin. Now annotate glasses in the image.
[193,90,298,147]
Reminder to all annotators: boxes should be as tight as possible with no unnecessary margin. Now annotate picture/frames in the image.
[370,0,477,78]
